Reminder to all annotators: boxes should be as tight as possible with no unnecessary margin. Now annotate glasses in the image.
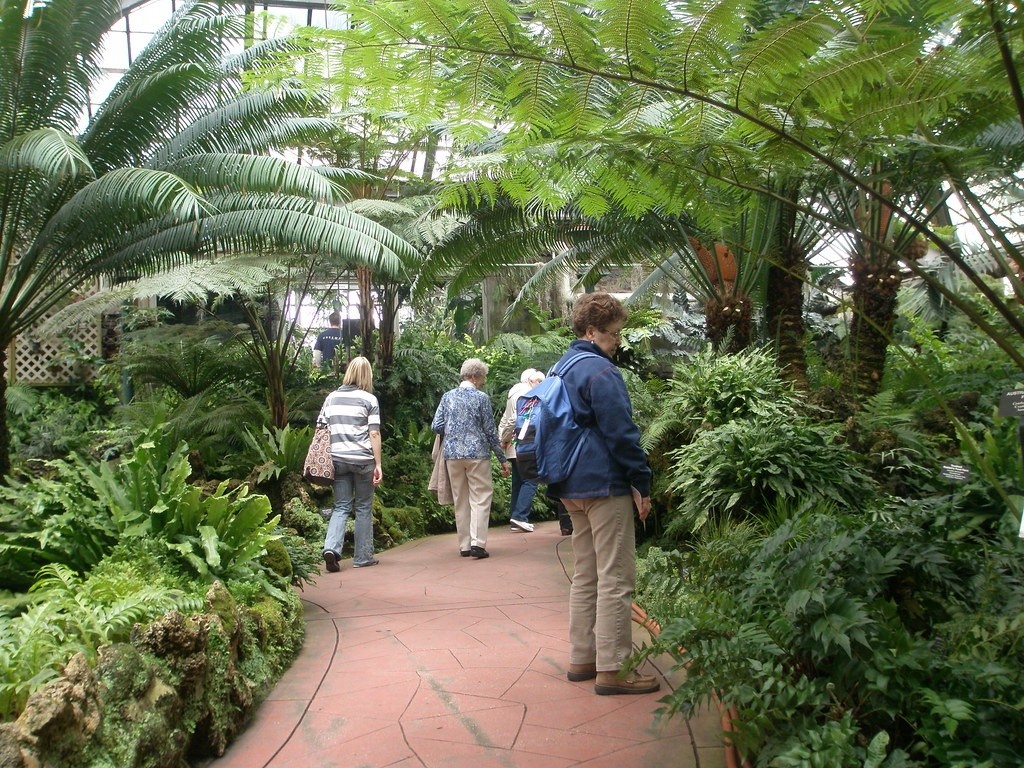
[603,327,621,341]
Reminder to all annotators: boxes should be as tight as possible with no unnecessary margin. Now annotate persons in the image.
[499,368,574,536]
[316,357,384,572]
[313,313,343,366]
[551,294,661,696]
[432,359,509,560]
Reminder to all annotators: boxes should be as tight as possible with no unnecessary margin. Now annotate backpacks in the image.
[512,351,605,484]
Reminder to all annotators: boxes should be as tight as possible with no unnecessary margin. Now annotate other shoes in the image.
[460,550,471,557]
[471,545,490,559]
[561,528,571,536]
[510,527,520,530]
[509,518,534,532]
[353,560,380,567]
[323,550,340,573]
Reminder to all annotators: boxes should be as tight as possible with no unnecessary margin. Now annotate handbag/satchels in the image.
[303,390,336,486]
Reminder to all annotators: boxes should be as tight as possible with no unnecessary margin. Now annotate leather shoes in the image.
[594,668,660,694]
[567,663,596,682]
